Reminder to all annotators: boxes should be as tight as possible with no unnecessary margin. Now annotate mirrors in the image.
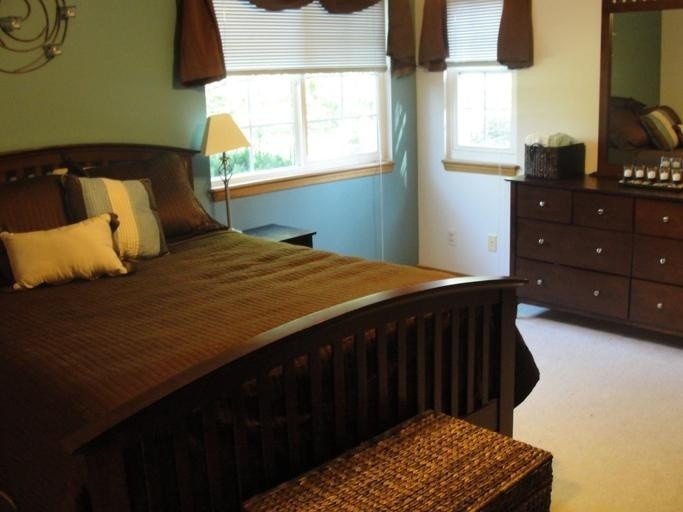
[597,0,683,179]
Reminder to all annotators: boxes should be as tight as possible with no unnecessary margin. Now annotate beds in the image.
[0,143,528,512]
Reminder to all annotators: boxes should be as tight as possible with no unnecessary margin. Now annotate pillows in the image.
[70,150,228,247]
[607,103,682,155]
[60,174,169,266]
[0,175,70,287]
[0,213,138,291]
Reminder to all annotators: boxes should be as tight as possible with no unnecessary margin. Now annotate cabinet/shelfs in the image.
[503,176,683,338]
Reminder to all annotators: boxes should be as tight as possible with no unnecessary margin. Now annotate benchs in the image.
[243,410,553,512]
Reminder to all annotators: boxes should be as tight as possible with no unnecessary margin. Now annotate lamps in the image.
[200,113,252,235]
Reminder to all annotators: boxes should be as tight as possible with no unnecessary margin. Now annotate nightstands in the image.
[241,224,317,249]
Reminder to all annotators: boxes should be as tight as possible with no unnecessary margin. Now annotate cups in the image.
[646,166,658,181]
[671,169,682,183]
[659,167,670,181]
[635,165,645,179]
[670,158,681,168]
[660,156,671,167]
[623,165,633,179]
